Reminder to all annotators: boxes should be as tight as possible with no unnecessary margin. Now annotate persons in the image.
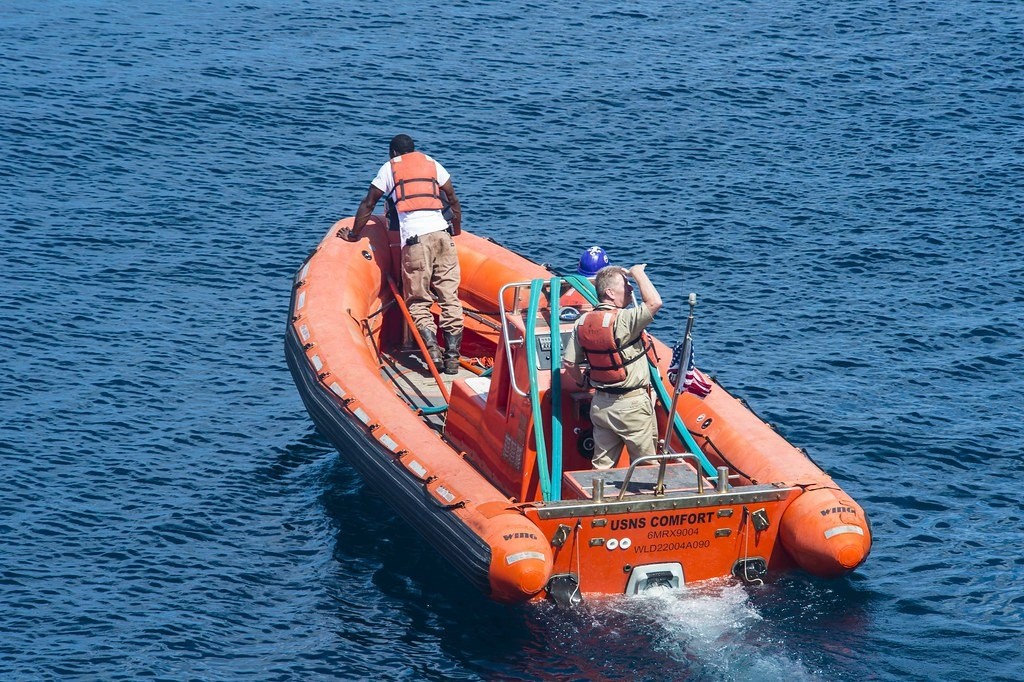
[563,264,662,470]
[336,134,464,374]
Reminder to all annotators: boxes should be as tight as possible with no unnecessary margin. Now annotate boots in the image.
[441,332,463,374]
[417,329,444,372]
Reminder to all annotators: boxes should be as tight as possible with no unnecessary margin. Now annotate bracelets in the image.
[349,231,357,239]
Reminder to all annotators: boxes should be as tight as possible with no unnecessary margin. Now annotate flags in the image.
[666,332,713,400]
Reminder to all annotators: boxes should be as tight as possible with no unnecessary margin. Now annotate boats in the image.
[285,213,871,606]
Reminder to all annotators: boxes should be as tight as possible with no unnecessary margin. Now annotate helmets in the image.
[577,246,611,276]
